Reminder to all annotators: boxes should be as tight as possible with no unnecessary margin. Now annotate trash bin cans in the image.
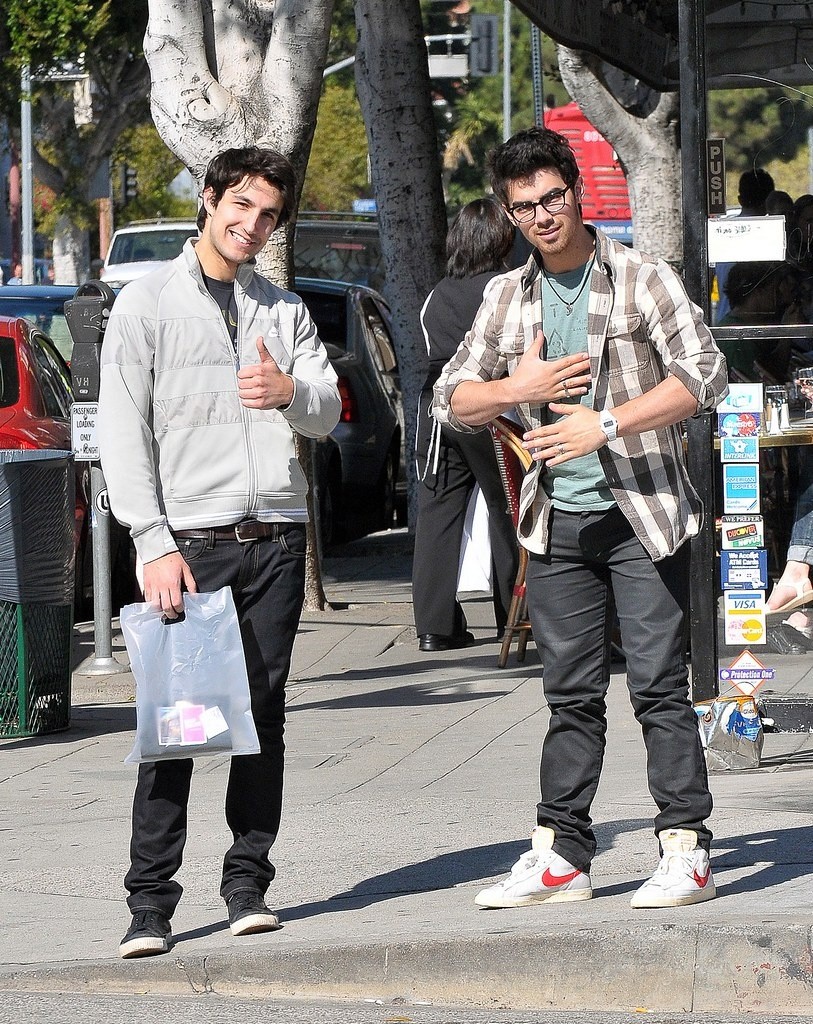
[0,449,76,739]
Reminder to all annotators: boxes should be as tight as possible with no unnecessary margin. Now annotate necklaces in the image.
[541,250,597,315]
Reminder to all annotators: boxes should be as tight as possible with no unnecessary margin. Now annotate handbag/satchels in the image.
[120,585,262,762]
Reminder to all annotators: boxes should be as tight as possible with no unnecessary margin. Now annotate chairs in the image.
[488,413,535,669]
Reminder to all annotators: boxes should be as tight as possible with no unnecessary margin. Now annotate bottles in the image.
[711,275,720,325]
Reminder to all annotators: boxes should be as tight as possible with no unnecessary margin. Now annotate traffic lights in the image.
[120,162,143,207]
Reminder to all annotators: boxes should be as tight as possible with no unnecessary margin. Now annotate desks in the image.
[678,419,813,450]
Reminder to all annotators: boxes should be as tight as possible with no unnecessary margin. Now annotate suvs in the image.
[91,218,201,294]
[0,281,141,614]
[292,207,390,300]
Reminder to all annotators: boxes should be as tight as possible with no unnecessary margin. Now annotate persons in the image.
[411,198,524,652]
[430,125,730,911]
[96,145,342,959]
[716,168,812,423]
[766,444,813,610]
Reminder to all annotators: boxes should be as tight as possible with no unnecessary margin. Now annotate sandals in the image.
[765,579,813,613]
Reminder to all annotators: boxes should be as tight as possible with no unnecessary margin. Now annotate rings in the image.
[562,382,568,389]
[565,390,570,396]
[556,444,564,455]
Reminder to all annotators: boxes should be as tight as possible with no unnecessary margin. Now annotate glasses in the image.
[507,186,570,223]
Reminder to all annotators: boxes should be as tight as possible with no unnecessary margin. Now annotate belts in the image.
[172,519,289,544]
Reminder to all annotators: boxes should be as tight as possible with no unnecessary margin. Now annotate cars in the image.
[0,314,93,622]
[293,272,409,542]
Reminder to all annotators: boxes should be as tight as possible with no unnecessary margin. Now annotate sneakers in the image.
[228,890,280,936]
[475,825,592,908]
[630,829,716,908]
[120,911,172,957]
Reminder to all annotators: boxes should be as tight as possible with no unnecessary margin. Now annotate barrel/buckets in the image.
[694,682,764,770]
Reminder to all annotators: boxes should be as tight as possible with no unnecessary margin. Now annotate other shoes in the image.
[420,632,475,651]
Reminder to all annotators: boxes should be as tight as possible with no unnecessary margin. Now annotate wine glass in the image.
[765,386,786,429]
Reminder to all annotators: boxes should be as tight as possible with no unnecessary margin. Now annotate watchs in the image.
[598,409,619,442]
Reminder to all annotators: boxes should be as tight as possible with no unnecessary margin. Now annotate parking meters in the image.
[61,276,133,677]
